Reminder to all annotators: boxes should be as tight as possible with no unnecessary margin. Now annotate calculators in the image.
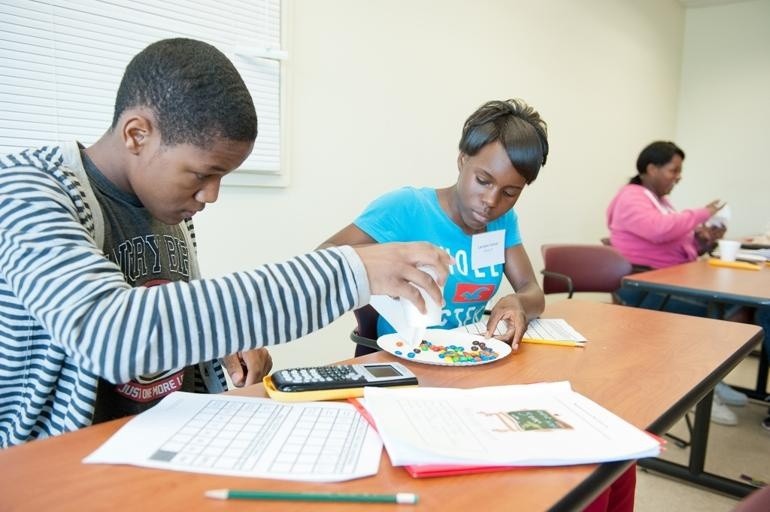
[265,361,418,400]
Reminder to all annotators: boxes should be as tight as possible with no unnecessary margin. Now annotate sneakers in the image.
[691,380,748,425]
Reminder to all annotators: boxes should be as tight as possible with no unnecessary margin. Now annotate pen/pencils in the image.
[523,339,587,349]
[206,489,418,504]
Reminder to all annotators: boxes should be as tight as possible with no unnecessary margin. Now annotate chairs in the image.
[539,238,651,306]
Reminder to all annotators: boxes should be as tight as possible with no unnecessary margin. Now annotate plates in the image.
[376,326,513,367]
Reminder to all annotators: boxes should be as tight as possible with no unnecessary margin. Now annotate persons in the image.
[608,141,727,268]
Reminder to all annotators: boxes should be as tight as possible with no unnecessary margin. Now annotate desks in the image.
[620,254,769,406]
[1,295,762,512]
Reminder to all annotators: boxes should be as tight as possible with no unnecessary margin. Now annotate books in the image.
[345,378,668,479]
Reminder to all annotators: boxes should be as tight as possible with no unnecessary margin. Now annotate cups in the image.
[716,238,741,263]
[403,266,446,326]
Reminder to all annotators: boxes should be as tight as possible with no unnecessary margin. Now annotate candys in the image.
[395,340,498,363]
